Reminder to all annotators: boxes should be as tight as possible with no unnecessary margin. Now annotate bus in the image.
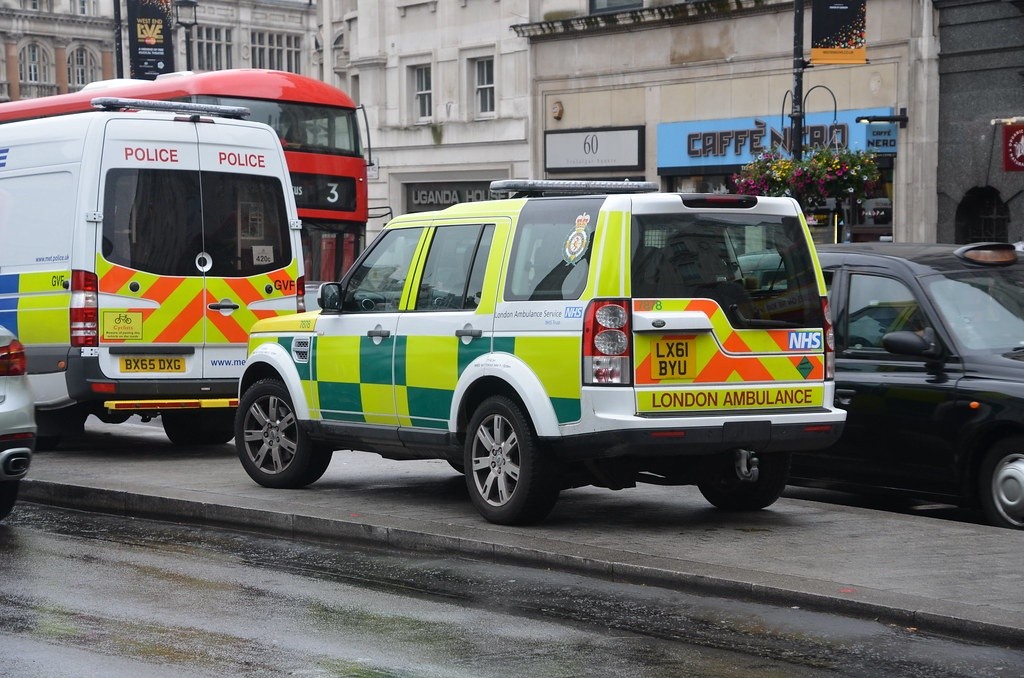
[0,69,393,314]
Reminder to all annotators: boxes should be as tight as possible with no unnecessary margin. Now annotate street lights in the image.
[174,0,199,72]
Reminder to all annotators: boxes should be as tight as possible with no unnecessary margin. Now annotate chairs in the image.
[444,245,488,309]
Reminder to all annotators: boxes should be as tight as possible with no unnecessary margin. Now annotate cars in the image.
[736,244,1024,530]
[0,325,35,518]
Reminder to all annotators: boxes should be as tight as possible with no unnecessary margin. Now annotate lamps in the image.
[856,108,908,128]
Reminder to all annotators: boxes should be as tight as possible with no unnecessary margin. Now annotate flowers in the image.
[732,142,881,208]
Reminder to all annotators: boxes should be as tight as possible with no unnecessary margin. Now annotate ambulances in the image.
[0,96,305,446]
[233,180,847,526]
